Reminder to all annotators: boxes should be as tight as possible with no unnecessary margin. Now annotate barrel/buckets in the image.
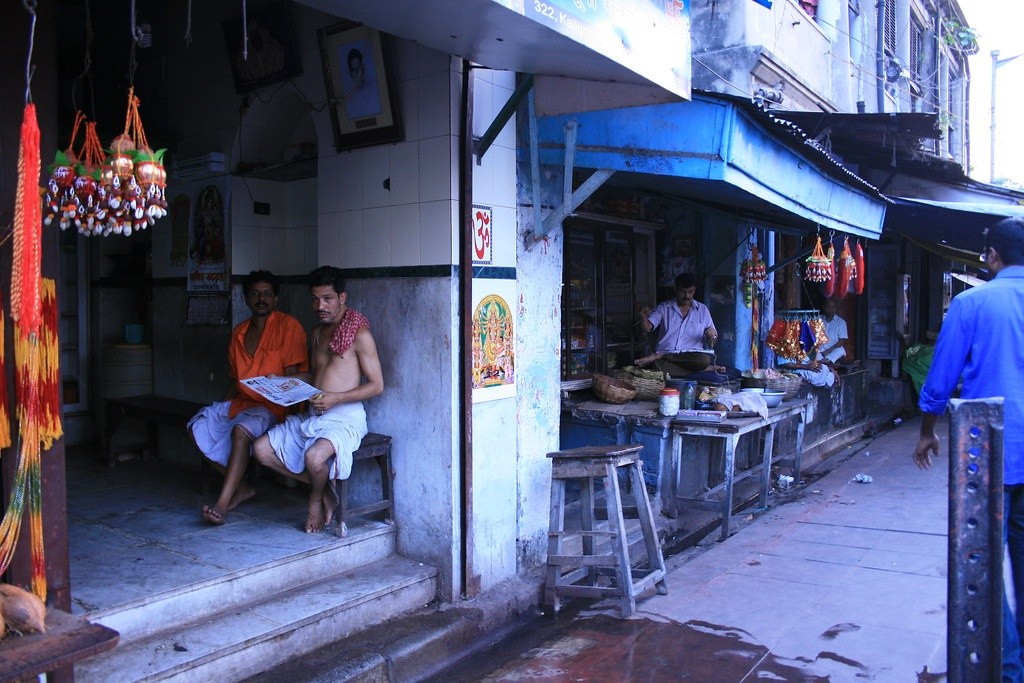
[665,379,698,409]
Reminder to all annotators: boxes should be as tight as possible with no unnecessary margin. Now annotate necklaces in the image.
[316,325,337,354]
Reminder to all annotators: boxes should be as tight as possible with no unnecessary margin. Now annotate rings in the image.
[815,365,817,366]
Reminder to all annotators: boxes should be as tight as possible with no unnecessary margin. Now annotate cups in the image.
[123,324,144,344]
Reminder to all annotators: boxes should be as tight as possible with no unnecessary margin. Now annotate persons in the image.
[912,217,1024,683]
[344,49,382,116]
[816,299,848,368]
[634,272,728,374]
[777,309,839,387]
[253,266,384,532]
[187,269,308,524]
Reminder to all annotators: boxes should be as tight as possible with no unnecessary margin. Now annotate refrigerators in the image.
[561,182,666,399]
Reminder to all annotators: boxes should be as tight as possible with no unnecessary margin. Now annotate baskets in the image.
[695,380,742,400]
[592,373,636,404]
[613,376,666,400]
[743,372,803,401]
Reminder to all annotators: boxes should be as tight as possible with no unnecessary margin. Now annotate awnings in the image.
[907,236,988,268]
[950,272,987,288]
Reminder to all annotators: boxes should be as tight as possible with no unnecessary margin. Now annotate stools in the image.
[317,20,406,153]
[544,446,668,616]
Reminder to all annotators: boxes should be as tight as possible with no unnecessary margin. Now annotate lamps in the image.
[785,0,902,84]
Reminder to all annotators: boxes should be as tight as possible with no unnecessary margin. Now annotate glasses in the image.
[979,250,988,262]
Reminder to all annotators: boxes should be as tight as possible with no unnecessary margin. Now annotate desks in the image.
[668,400,809,539]
[0,606,120,683]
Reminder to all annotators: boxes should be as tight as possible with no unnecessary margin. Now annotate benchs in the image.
[334,432,396,538]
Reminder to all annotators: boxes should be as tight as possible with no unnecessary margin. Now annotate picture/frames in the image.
[673,233,697,282]
[218,0,303,96]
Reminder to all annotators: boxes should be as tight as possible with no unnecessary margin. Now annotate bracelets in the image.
[714,336,716,338]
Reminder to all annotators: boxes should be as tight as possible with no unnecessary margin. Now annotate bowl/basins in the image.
[741,388,786,408]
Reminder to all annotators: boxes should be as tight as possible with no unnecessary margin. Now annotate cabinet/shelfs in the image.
[562,288,633,379]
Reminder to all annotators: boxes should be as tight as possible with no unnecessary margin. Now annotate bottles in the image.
[684,383,694,409]
[659,389,680,416]
